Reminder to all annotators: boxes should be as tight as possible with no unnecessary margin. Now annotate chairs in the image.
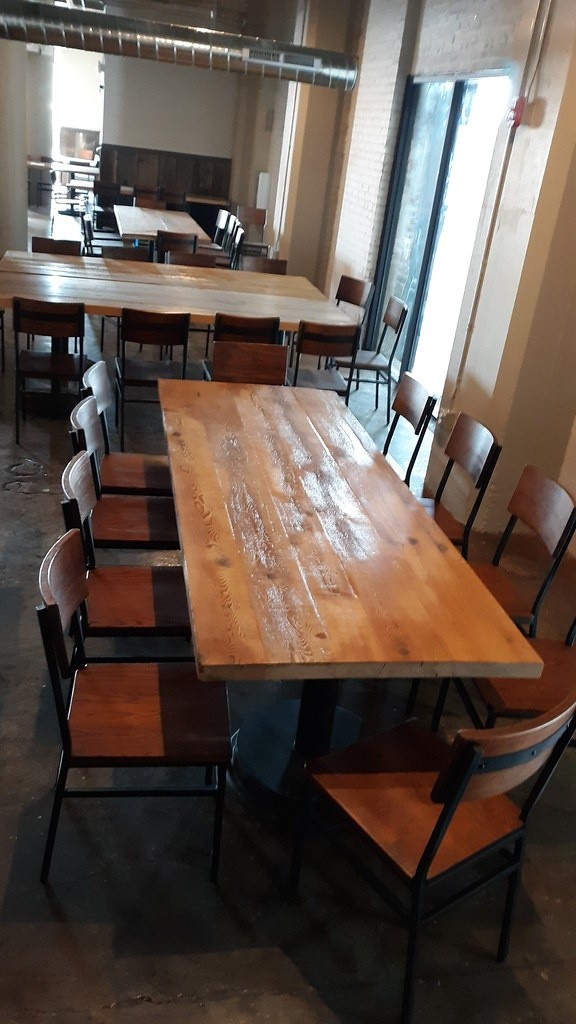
[115,308,191,455]
[35,527,232,886]
[289,319,362,408]
[329,295,408,425]
[80,360,168,458]
[283,688,576,1024]
[399,463,575,732]
[26,152,286,358]
[288,275,374,378]
[430,620,576,793]
[9,296,88,444]
[201,312,289,388]
[379,370,439,486]
[413,412,502,563]
[61,449,195,657]
[66,388,181,560]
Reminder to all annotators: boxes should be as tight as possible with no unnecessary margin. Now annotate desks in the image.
[27,160,99,216]
[113,205,211,247]
[0,248,352,415]
[157,378,543,848]
